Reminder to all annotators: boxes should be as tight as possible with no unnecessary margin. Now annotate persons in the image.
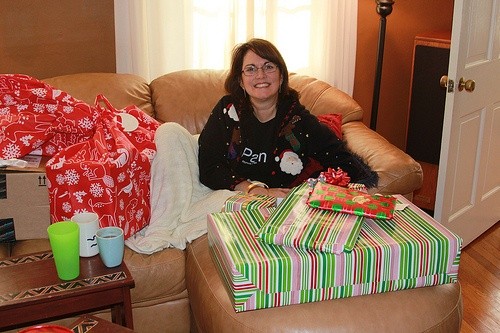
[195,37,380,201]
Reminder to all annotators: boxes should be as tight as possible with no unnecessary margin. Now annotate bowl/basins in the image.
[18,324,75,333]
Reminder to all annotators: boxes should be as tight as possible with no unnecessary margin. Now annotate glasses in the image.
[241,61,279,76]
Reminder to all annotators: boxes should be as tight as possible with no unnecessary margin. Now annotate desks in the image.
[0,246,135,330]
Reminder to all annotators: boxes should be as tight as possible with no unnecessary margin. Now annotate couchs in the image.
[0,69,463,333]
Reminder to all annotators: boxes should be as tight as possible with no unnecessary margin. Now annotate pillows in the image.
[287,113,343,186]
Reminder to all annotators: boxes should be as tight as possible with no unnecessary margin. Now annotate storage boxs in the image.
[0,155,51,239]
[224,191,277,209]
[209,194,463,312]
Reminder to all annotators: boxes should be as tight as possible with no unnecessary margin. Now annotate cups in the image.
[46,220,80,280]
[95,227,124,268]
[70,212,100,257]
